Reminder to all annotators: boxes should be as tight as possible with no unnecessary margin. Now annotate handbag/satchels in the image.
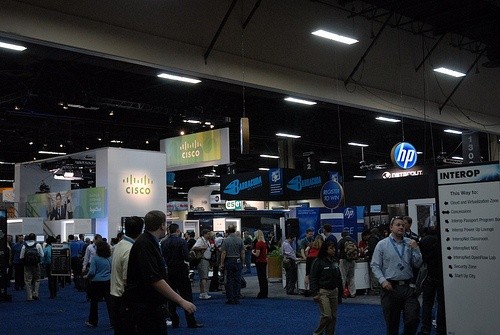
[282,259,290,268]
[193,248,203,262]
[415,265,439,290]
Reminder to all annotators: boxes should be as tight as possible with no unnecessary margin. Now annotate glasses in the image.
[395,216,402,219]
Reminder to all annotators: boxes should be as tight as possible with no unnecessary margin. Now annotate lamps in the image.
[53,169,83,182]
[156,71,202,84]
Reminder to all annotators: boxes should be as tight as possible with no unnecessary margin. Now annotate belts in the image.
[398,280,405,286]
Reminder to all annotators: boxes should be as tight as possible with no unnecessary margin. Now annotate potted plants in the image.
[268,244,282,282]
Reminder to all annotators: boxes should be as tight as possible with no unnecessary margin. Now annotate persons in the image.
[310,240,338,335]
[337,232,358,298]
[122,210,196,335]
[48,193,73,220]
[161,223,205,328]
[184,232,196,253]
[418,223,450,335]
[251,230,269,299]
[370,216,423,335]
[301,229,324,297]
[243,231,253,274]
[7,232,125,327]
[191,229,211,300]
[359,223,391,295]
[210,233,226,292]
[323,224,343,303]
[282,233,298,295]
[402,216,418,244]
[110,216,144,335]
[221,226,246,304]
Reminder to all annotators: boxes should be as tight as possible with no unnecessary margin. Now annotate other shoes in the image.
[226,299,240,305]
[85,321,93,327]
[199,292,211,299]
[188,323,203,327]
[209,287,222,292]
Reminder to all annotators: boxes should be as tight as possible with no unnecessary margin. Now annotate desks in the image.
[282,260,370,293]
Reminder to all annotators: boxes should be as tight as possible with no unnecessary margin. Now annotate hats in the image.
[201,229,210,235]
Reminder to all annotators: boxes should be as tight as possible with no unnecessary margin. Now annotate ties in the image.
[58,209,61,219]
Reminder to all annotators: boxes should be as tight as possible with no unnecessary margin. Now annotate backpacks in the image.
[23,241,40,267]
[345,241,358,259]
